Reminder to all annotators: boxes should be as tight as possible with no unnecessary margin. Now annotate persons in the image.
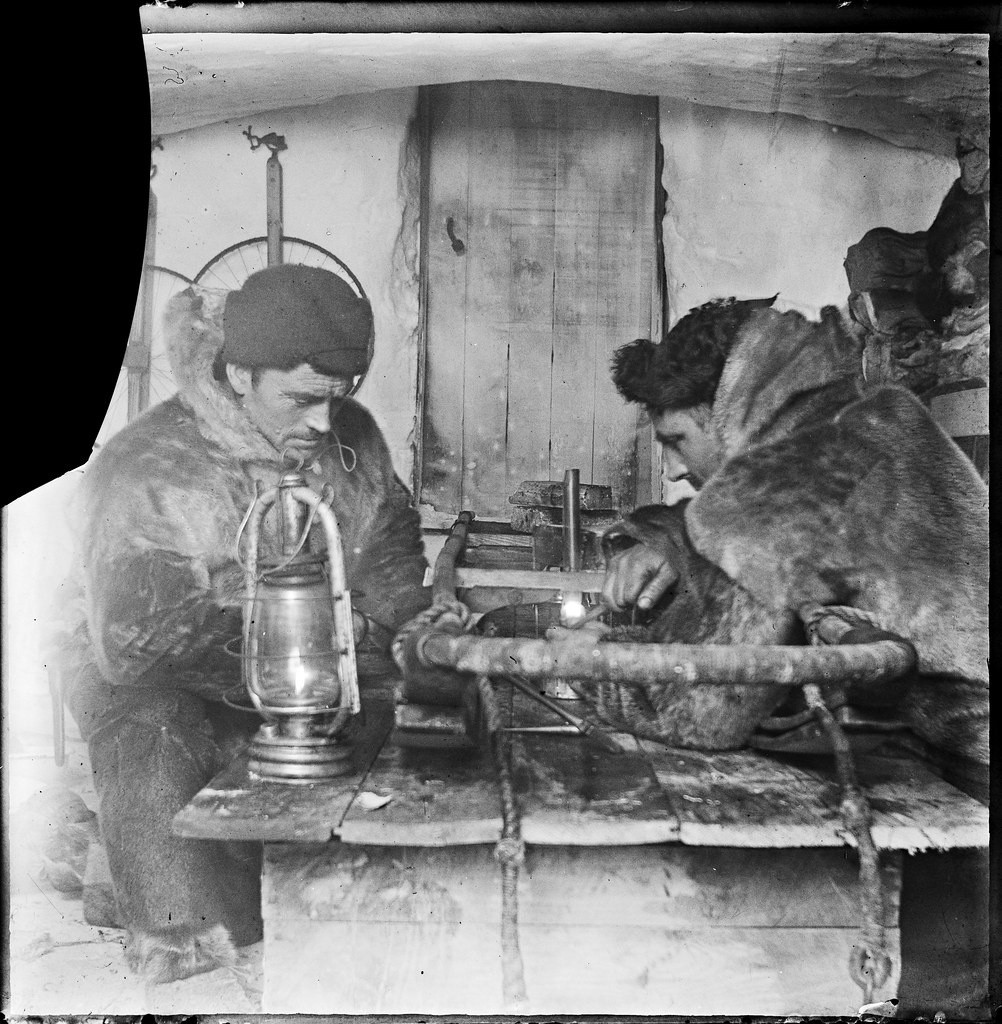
[546,298,990,1008]
[58,264,424,983]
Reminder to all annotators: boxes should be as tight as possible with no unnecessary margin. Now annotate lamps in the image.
[229,473,366,787]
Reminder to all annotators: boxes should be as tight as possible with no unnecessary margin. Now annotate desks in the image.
[174,503,1002,1017]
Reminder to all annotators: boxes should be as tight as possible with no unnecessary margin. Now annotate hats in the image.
[211,262,376,382]
